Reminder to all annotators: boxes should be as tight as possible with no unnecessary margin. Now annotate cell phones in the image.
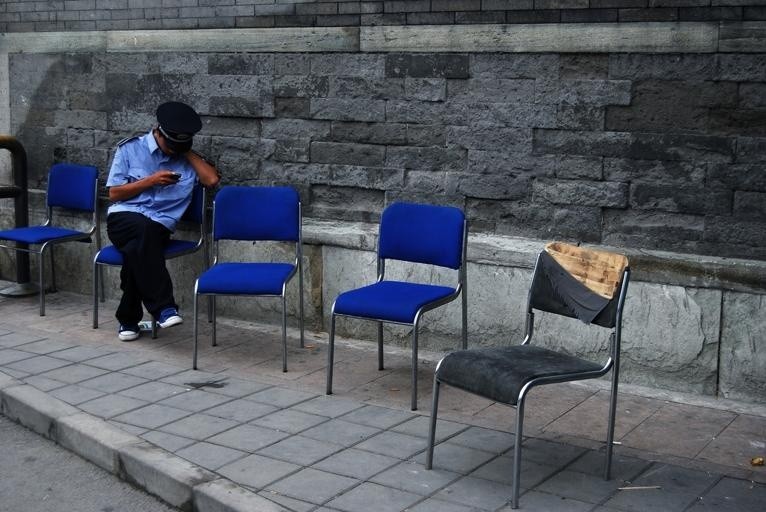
[169,172,182,180]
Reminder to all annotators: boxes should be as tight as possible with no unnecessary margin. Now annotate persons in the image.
[105,101,220,342]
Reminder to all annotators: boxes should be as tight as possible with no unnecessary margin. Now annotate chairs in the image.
[1,161,104,316]
[193,186,304,372]
[92,185,212,338]
[326,202,467,411]
[426,242,629,510]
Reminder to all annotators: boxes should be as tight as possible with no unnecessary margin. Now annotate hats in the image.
[155,101,203,153]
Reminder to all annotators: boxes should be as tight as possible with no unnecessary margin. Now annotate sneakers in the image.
[156,307,183,329]
[118,323,141,342]
